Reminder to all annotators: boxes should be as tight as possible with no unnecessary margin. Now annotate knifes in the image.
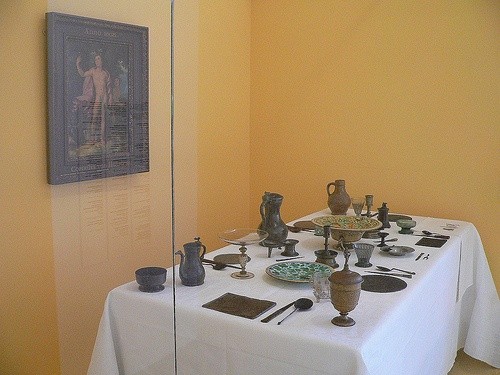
[416,253,424,260]
[415,235,450,238]
[365,270,413,279]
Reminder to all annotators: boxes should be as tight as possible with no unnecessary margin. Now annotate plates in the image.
[380,245,415,256]
[265,261,334,283]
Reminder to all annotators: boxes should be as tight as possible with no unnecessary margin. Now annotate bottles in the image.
[326,180,351,215]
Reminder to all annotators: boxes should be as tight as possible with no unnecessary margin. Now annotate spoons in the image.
[277,298,313,325]
[376,266,415,275]
[422,231,445,237]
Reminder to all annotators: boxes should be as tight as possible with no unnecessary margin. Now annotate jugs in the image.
[256,192,289,248]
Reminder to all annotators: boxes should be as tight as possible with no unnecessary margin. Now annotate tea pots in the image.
[175,237,206,286]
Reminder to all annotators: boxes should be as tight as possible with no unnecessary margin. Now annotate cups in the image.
[351,197,366,216]
[354,243,375,268]
[312,270,334,303]
[135,266,167,293]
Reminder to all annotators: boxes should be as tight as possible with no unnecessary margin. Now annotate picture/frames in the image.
[45,12,150,185]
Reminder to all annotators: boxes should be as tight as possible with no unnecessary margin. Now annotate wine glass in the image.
[328,269,365,327]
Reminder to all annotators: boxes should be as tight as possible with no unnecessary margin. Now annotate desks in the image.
[86,207,500,375]
